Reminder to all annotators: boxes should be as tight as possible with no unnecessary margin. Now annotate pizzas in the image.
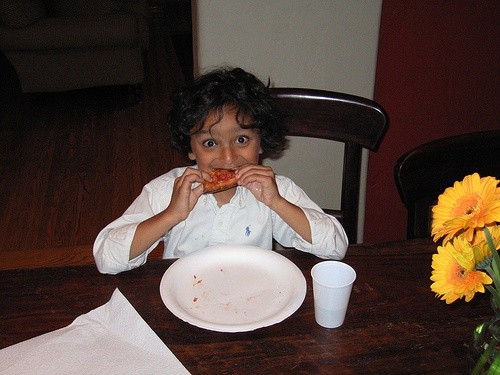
[203,168,238,194]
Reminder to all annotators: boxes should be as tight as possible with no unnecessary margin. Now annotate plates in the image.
[160,245,307,332]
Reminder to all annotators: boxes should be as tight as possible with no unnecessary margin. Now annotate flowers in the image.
[426,172,500,318]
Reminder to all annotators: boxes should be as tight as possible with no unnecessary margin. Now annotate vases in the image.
[472,319,500,375]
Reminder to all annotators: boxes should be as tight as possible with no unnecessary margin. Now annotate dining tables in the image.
[0,235,500,374]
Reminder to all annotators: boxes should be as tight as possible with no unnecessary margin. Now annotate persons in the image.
[94,67,348,274]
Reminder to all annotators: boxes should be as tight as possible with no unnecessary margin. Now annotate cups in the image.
[311,261,356,328]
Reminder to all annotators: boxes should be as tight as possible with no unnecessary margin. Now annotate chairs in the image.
[393,129,500,239]
[258,87,390,251]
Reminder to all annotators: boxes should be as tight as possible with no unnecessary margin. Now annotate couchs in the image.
[0,0,150,104]
